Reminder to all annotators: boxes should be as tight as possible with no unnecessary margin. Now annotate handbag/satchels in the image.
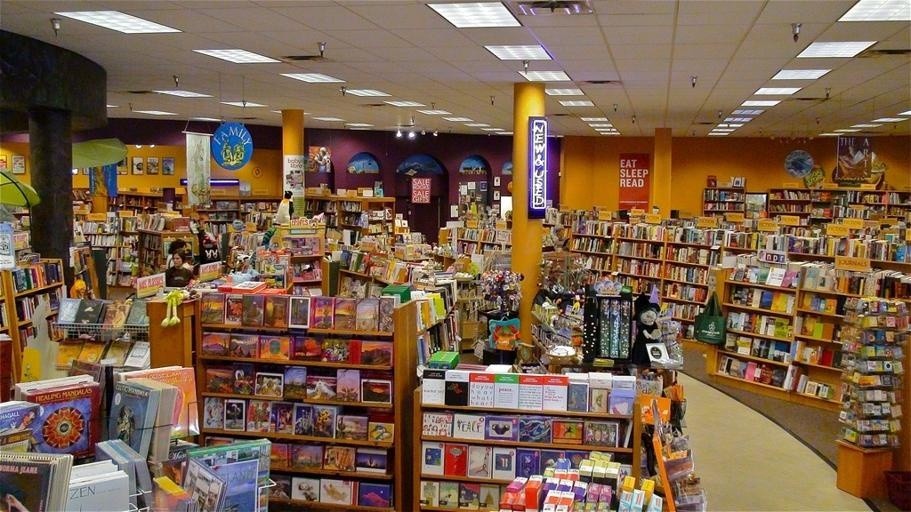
[692,290,727,345]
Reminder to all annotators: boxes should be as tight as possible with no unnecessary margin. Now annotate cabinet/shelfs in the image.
[694,188,911,407]
[831,289,911,498]
[1,187,700,512]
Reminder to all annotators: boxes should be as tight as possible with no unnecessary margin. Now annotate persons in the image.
[231,144,244,166]
[147,161,159,174]
[136,163,143,174]
[314,147,330,173]
[221,142,234,165]
[164,162,171,173]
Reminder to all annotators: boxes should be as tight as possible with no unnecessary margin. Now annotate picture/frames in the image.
[0,154,26,174]
[116,156,175,175]
